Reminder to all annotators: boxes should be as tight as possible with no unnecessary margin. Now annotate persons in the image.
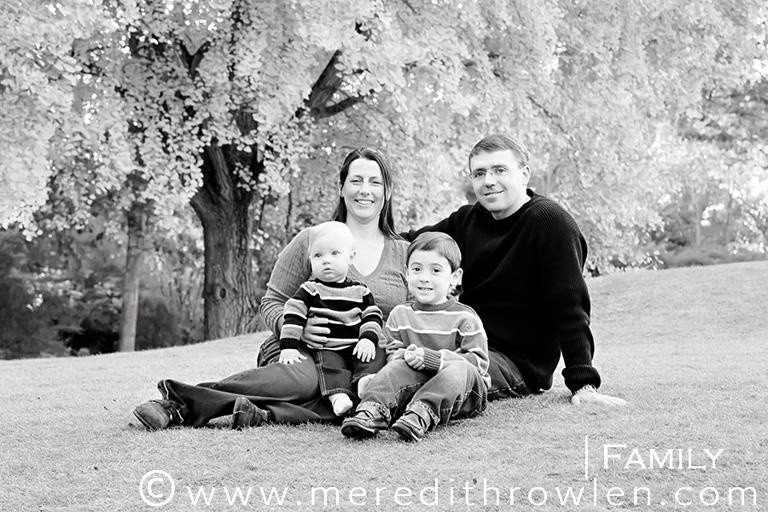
[134,146,411,430]
[341,231,490,442]
[400,133,628,409]
[278,219,386,415]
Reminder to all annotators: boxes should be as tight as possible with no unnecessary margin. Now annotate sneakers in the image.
[340,411,388,437]
[391,411,426,441]
[232,396,268,429]
[133,399,177,430]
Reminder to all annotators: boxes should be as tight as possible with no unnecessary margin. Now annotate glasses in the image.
[469,165,518,181]
